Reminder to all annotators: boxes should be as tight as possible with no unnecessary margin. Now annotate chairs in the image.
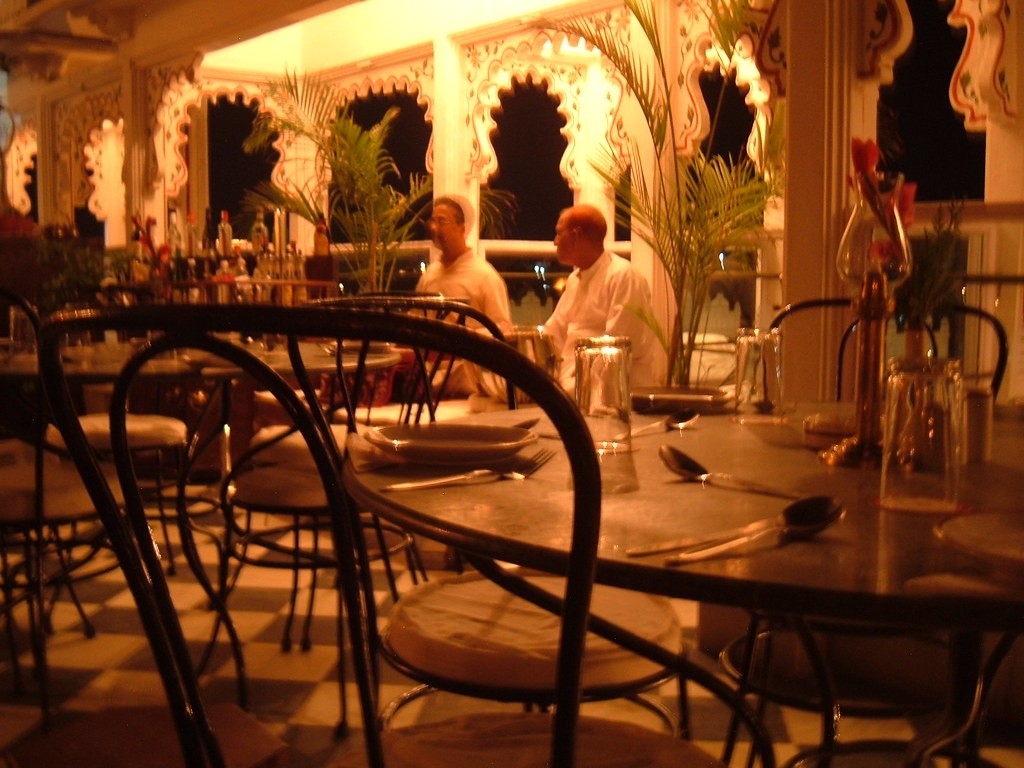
[0,287,1024,768]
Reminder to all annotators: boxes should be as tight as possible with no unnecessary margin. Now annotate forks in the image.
[663,504,845,562]
[380,446,557,492]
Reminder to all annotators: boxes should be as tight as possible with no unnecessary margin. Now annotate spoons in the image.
[512,418,541,430]
[612,411,698,441]
[625,496,842,557]
[658,445,805,501]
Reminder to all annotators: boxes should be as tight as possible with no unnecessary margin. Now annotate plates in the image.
[362,422,538,462]
[940,515,1023,565]
[334,338,393,352]
[629,386,728,415]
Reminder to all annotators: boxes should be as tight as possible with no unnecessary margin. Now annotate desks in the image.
[346,407,1024,768]
[0,341,392,711]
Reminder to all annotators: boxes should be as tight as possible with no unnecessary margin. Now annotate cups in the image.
[574,336,631,455]
[733,327,782,422]
[474,323,559,402]
[880,356,970,512]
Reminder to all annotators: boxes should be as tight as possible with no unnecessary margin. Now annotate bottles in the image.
[7,298,105,349]
[40,205,306,307]
[314,211,330,264]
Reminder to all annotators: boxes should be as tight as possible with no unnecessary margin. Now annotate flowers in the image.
[848,138,962,330]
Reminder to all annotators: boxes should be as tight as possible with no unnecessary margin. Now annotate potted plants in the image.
[242,65,515,312]
[526,0,798,416]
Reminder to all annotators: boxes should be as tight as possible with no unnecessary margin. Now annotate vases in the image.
[888,332,944,461]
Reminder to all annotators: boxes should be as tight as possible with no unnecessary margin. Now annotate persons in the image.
[416,197,511,335]
[544,205,667,389]
